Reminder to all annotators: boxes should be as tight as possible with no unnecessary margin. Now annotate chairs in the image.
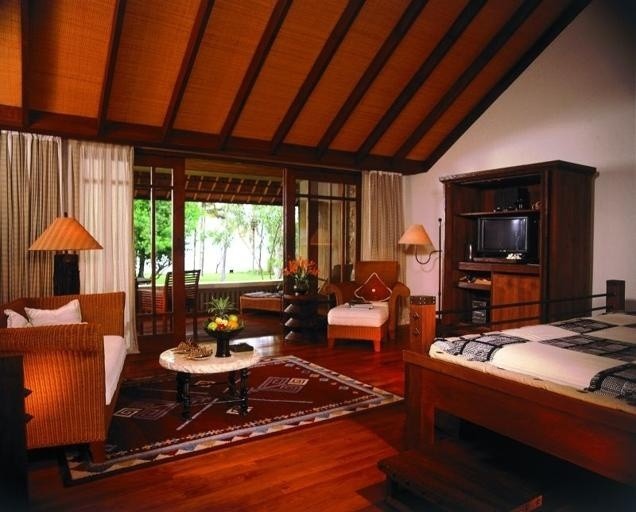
[138,265,202,338]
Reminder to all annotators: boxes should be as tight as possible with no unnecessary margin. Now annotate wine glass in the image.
[203,322,245,358]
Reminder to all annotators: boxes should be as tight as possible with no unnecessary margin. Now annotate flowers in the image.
[280,258,322,279]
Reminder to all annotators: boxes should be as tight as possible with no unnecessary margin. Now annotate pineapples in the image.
[219,314,228,320]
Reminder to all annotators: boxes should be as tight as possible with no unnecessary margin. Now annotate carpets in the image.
[56,354,408,489]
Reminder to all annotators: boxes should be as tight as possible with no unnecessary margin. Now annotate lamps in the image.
[396,216,442,324]
[25,217,107,295]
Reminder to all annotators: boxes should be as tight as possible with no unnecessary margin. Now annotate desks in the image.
[135,275,153,339]
[236,288,283,325]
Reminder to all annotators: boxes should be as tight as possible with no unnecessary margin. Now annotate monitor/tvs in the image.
[477,216,528,253]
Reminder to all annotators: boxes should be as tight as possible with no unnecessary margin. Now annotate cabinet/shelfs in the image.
[0,352,37,512]
[438,157,597,335]
[283,293,320,345]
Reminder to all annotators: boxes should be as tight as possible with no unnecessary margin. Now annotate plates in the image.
[189,355,210,360]
[171,349,188,354]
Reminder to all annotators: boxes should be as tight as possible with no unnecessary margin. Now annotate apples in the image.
[208,322,217,331]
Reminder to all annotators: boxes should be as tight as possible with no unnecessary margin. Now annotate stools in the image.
[373,440,545,511]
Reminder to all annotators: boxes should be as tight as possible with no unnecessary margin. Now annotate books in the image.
[229,343,253,352]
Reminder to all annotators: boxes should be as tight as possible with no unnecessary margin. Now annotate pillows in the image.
[353,271,394,306]
[21,298,84,325]
[2,306,30,326]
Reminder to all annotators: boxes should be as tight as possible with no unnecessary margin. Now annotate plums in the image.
[217,324,225,331]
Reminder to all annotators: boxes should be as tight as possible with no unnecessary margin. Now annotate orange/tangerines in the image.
[229,315,238,322]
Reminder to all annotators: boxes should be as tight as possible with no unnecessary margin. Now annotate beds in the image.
[405,278,634,491]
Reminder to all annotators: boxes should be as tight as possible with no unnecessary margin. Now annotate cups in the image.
[466,241,476,262]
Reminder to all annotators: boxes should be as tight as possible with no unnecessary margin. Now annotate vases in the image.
[292,275,312,292]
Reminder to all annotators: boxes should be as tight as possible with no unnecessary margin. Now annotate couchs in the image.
[319,259,411,354]
[0,291,129,466]
